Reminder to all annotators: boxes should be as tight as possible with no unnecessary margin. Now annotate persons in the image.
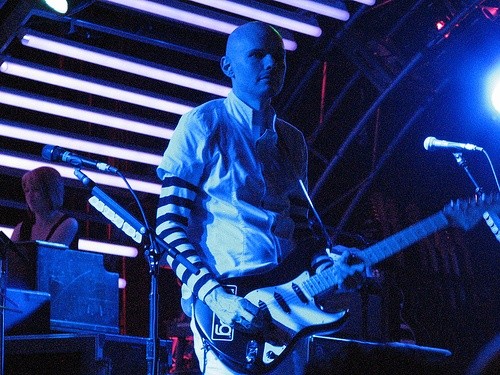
[156,21,374,375]
[10,166,79,246]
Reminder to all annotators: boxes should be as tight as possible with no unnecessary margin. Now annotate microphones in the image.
[424,136,483,154]
[41,144,118,175]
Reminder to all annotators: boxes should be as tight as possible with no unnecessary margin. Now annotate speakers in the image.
[99,332,173,375]
[306,335,453,375]
[3,288,50,336]
[4,334,96,375]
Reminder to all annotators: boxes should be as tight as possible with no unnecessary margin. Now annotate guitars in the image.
[193,188,499,375]
